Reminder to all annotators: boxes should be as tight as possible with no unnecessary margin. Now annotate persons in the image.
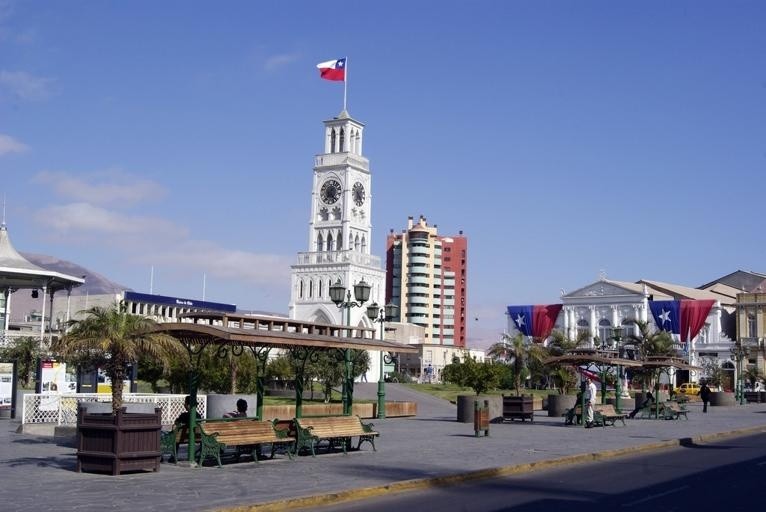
[585,376,593,427]
[626,390,653,418]
[223,398,250,417]
[697,378,711,413]
[168,396,203,463]
[568,380,585,426]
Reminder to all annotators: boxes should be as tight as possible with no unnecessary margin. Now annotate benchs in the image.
[562,400,690,427]
[161,414,378,467]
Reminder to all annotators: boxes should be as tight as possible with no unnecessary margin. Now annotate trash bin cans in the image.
[542,399,549,410]
[605,398,617,407]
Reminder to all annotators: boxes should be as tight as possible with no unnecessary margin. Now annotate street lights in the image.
[609,324,627,413]
[730,345,751,405]
[593,337,614,426]
[366,297,399,420]
[329,277,371,451]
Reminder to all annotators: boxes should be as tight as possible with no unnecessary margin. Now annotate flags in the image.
[315,58,345,81]
[504,302,562,340]
[647,300,719,339]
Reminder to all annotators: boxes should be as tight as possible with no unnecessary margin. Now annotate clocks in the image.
[352,182,365,205]
[320,180,341,204]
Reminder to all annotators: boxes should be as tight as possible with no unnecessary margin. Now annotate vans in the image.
[674,382,700,395]
